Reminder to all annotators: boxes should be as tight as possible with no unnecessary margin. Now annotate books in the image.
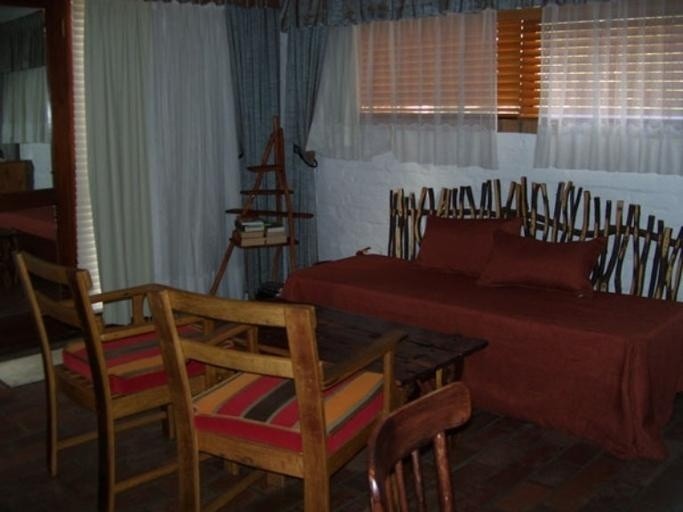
[232,216,287,246]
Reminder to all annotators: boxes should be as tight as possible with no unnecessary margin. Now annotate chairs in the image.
[370,382,472,512]
[13,248,235,507]
[148,287,409,509]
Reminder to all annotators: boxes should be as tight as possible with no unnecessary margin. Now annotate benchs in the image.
[282,178,679,455]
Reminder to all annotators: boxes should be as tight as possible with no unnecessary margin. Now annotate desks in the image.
[201,297,488,433]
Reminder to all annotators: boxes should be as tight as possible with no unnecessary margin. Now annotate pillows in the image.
[411,213,610,293]
